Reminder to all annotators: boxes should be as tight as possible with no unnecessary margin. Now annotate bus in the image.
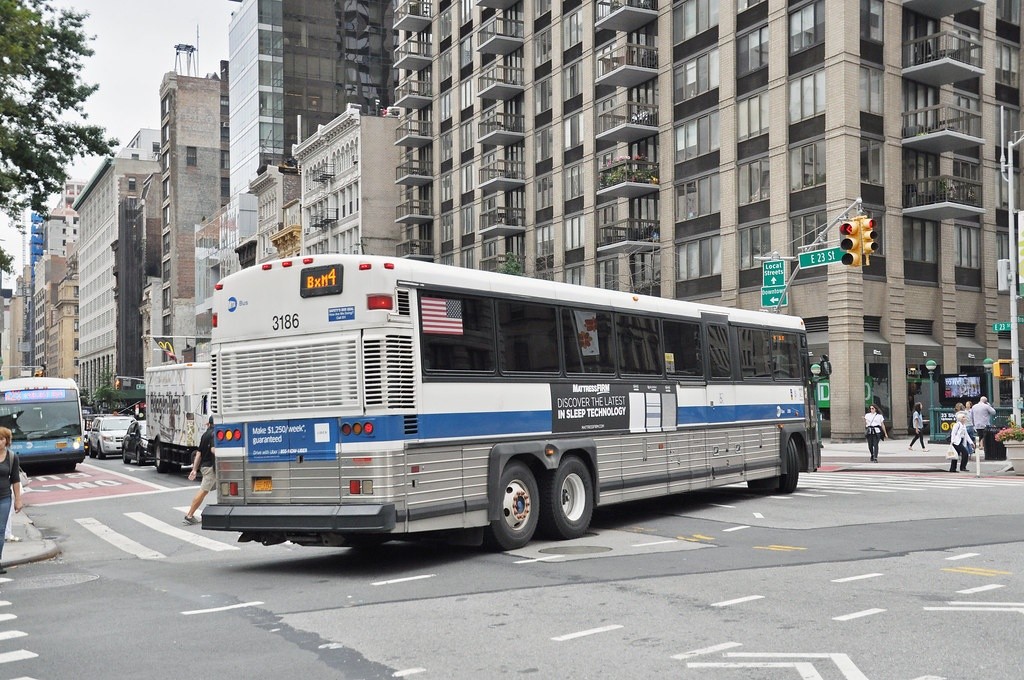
[200,253,832,551]
[1,376,86,474]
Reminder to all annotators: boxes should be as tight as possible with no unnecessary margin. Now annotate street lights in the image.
[924,359,938,443]
[982,356,995,427]
[810,364,824,449]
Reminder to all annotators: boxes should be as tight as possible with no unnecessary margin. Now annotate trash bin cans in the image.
[984,425,1006,460]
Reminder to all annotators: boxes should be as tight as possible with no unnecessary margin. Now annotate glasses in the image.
[870,407,875,410]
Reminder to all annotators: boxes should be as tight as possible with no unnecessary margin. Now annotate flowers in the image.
[601,155,653,167]
[995,423,1024,441]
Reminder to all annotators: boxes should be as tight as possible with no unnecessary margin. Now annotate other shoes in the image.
[922,447,929,451]
[950,469,959,473]
[5,535,23,541]
[960,468,970,471]
[183,516,198,524]
[873,458,878,463]
[0,563,8,574]
[870,455,874,461]
[909,447,915,450]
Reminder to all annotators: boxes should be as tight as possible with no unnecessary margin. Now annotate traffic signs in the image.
[759,261,790,308]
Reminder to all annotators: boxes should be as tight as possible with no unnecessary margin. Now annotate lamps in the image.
[923,352,928,356]
[873,350,882,355]
[807,351,813,357]
[968,354,976,359]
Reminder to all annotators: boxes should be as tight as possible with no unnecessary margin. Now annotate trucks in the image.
[144,361,211,476]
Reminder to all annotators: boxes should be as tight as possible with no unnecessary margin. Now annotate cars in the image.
[82,413,139,460]
[122,420,155,466]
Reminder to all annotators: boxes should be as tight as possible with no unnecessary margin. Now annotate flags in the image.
[153,337,177,363]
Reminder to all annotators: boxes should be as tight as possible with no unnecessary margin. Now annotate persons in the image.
[114,412,118,416]
[0,427,23,574]
[864,405,888,462]
[950,396,996,472]
[908,402,929,451]
[184,415,215,524]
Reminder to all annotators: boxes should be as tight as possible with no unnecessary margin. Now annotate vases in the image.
[1003,440,1024,476]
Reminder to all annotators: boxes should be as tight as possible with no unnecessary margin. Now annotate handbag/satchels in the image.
[946,445,958,460]
[19,465,29,486]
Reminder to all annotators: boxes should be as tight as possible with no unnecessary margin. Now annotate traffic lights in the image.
[856,214,879,254]
[836,218,864,267]
[993,362,1011,378]
